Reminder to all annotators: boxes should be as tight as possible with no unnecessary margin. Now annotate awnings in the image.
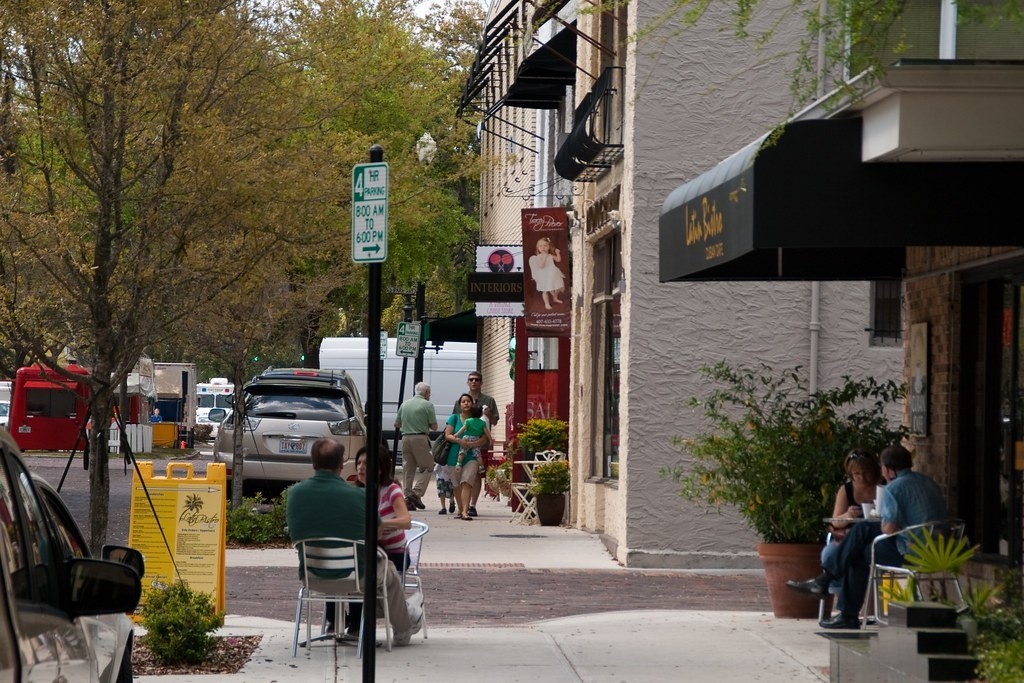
[657,120,1024,283]
[429,308,477,342]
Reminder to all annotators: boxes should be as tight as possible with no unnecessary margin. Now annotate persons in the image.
[285,438,422,646]
[433,463,456,515]
[444,394,487,520]
[149,408,162,422]
[454,404,494,473]
[527,238,566,310]
[394,382,437,510]
[452,371,499,516]
[820,446,886,614]
[323,444,411,638]
[786,444,947,628]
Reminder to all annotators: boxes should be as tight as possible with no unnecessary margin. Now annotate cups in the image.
[862,503,874,518]
[876,483,886,516]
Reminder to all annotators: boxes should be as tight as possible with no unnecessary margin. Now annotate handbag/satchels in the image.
[431,431,452,466]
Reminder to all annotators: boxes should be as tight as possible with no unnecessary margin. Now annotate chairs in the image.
[507,448,567,527]
[814,516,970,633]
[292,520,430,659]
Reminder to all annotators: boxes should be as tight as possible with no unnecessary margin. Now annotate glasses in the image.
[467,378,480,382]
[849,452,870,460]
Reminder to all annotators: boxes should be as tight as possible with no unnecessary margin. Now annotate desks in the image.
[513,460,563,527]
[823,517,885,545]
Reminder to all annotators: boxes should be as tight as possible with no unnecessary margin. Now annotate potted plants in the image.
[653,353,928,620]
[486,418,574,526]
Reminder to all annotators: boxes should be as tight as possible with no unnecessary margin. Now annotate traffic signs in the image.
[350,161,388,263]
[396,321,422,357]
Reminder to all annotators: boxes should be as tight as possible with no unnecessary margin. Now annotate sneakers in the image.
[393,607,424,646]
[401,592,424,618]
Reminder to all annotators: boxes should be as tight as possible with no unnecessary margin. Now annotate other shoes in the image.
[453,512,461,519]
[403,497,416,511]
[454,462,462,472]
[408,494,425,510]
[478,465,485,473]
[449,501,455,513]
[439,508,447,514]
[340,633,360,642]
[326,619,349,633]
[468,506,477,516]
[461,514,470,520]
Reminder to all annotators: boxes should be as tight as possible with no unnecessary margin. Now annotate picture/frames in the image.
[905,319,931,438]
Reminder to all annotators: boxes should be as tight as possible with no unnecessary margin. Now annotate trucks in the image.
[194,377,234,438]
[0,380,12,429]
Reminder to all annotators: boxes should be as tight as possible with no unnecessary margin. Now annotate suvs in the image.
[213,364,388,499]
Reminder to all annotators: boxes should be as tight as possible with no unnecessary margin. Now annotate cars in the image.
[0,422,146,683]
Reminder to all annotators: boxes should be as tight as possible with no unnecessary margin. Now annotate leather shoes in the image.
[785,577,828,600]
[819,612,860,629]
